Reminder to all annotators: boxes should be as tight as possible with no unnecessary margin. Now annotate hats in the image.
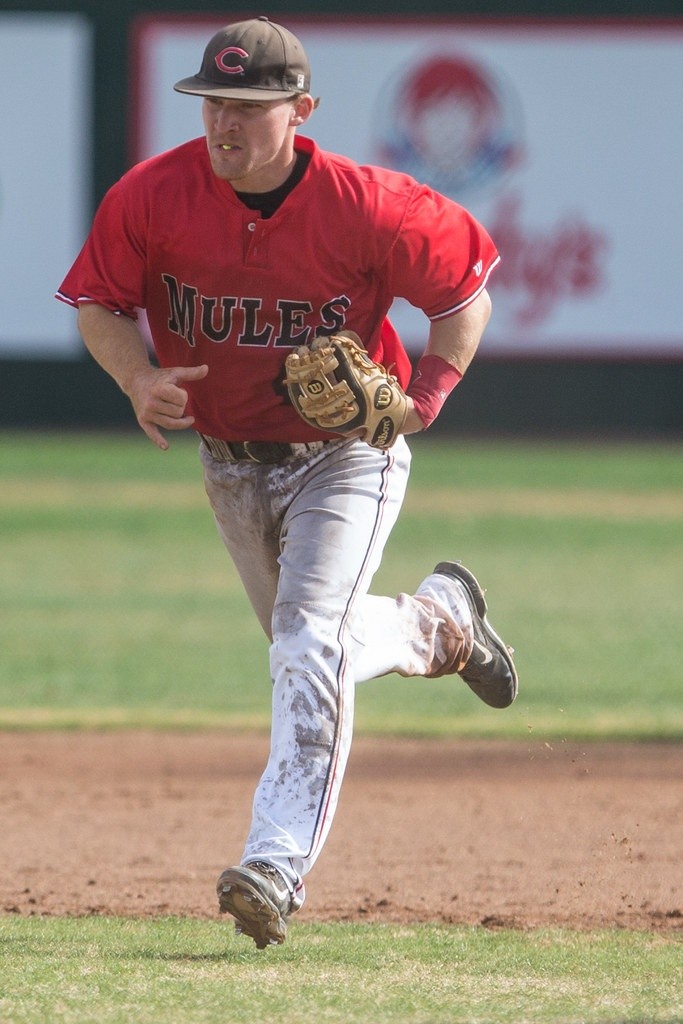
[173,16,311,102]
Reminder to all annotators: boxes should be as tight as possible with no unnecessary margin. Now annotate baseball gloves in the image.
[282,329,409,452]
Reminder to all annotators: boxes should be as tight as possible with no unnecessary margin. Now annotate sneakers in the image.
[216,860,291,949]
[434,561,518,709]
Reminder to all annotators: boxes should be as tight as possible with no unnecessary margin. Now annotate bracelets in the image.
[408,356,464,430]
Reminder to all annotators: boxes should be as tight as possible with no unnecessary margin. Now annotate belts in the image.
[195,430,331,463]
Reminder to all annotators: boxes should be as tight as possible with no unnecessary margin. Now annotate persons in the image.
[54,14,518,950]
